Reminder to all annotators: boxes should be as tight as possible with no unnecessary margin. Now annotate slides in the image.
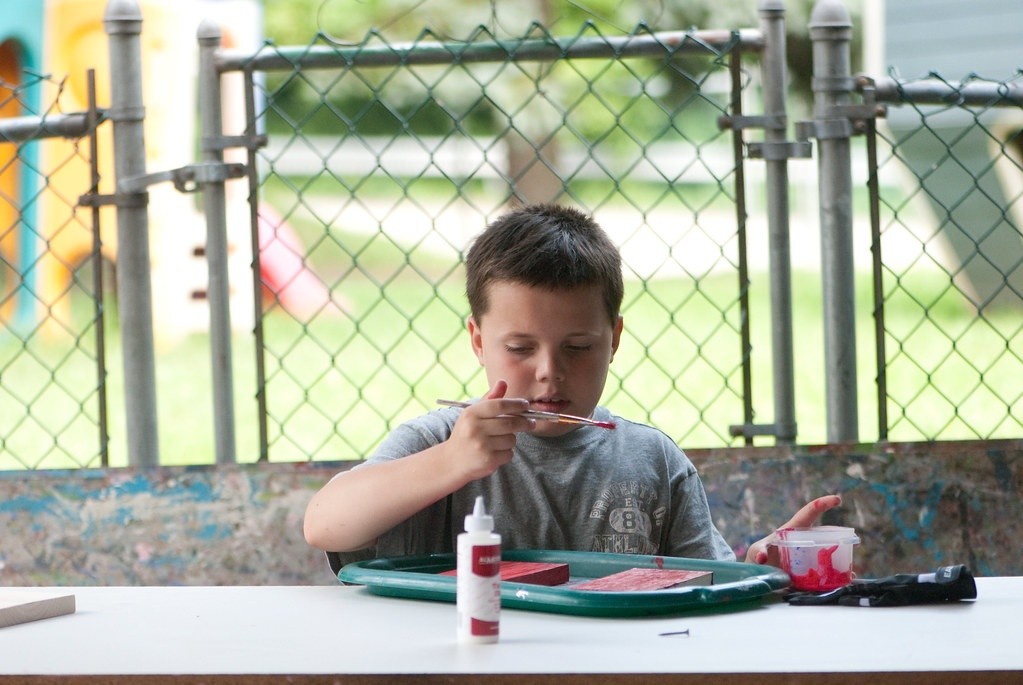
[256,200,358,317]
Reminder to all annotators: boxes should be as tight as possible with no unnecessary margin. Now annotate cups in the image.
[771,525,861,592]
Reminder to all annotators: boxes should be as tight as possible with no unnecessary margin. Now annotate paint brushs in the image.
[435,398,618,430]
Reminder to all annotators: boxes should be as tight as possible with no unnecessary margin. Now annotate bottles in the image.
[457,496,501,645]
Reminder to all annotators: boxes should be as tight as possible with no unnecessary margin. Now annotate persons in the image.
[302,203,840,586]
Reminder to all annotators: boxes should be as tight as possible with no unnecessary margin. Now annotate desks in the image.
[0,576,1023,685]
